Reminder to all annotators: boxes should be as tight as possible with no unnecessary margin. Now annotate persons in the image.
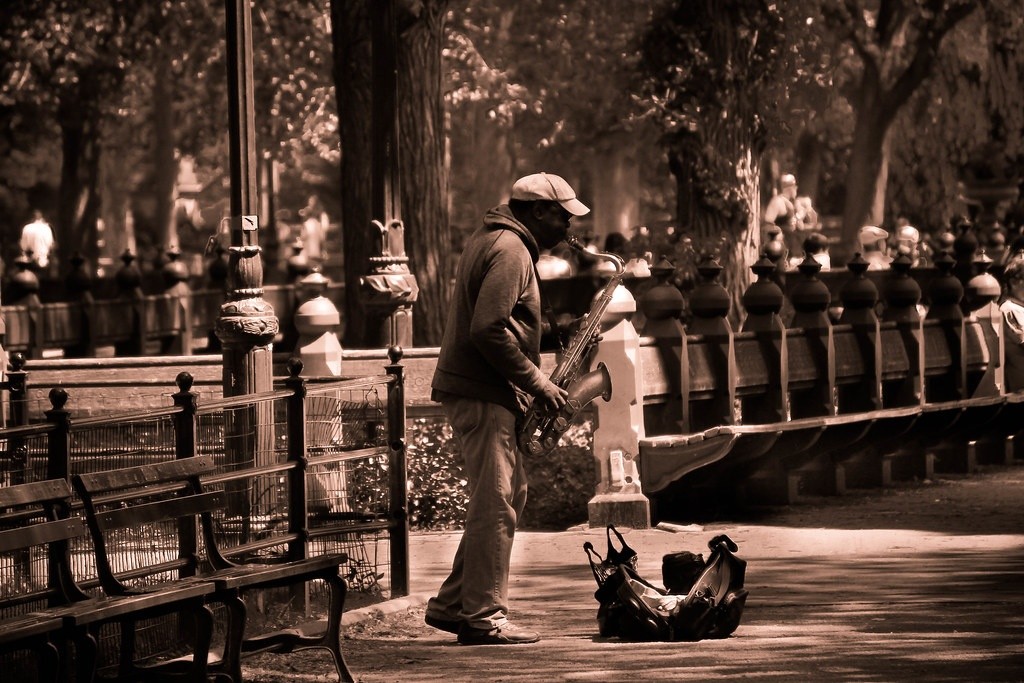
[273,193,329,276]
[1000,252,1024,391]
[536,230,692,281]
[19,210,53,282]
[760,174,1024,268]
[450,224,466,277]
[426,173,603,646]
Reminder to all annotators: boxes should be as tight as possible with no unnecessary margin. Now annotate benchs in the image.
[73,451,355,683]
[0,477,219,683]
[640,392,1024,506]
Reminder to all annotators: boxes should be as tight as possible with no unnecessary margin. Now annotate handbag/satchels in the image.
[593,535,748,641]
[583,524,638,588]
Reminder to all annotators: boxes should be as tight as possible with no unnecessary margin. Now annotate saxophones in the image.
[514,234,627,458]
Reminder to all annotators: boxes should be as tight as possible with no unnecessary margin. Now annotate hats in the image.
[511,172,590,217]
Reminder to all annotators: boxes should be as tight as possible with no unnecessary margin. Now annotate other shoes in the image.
[459,621,542,644]
[425,613,461,635]
